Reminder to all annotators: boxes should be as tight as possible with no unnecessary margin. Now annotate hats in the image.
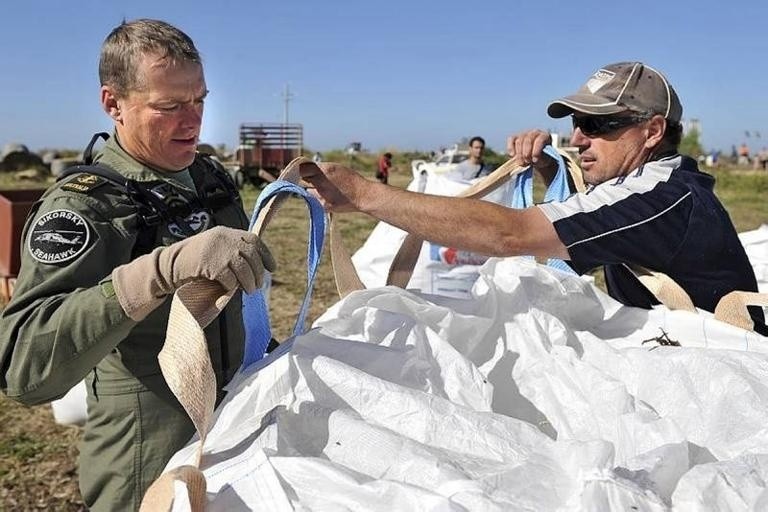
[548,62,682,122]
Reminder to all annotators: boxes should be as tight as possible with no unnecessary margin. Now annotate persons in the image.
[313,152,323,163]
[757,145,767,171]
[0,17,281,512]
[457,136,493,182]
[729,144,738,167]
[751,153,760,170]
[736,142,751,167]
[296,61,767,337]
[710,148,722,169]
[379,153,393,185]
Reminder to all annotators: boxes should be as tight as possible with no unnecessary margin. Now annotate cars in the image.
[412,151,487,180]
[210,155,242,188]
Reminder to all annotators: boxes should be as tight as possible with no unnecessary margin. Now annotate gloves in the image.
[111,226,276,321]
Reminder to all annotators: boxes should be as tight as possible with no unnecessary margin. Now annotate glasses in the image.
[572,115,650,134]
